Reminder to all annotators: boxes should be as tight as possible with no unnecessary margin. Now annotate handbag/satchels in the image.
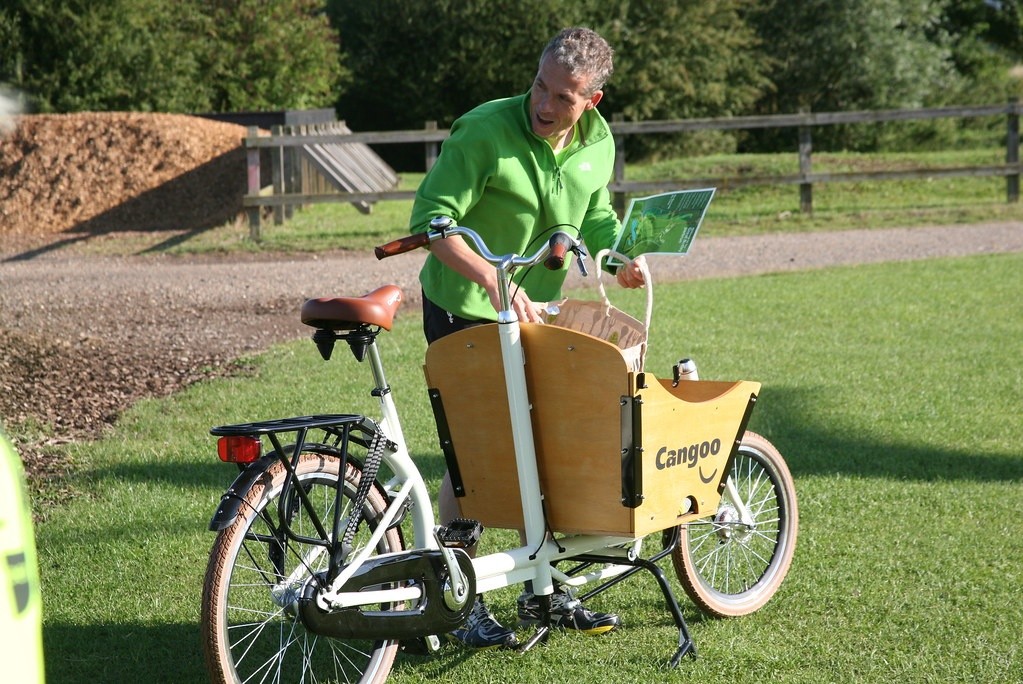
[524,248,653,373]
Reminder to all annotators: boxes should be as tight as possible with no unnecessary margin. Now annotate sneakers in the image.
[445,592,520,650]
[517,588,620,636]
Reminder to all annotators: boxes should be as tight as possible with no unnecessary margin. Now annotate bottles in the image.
[679,359,699,381]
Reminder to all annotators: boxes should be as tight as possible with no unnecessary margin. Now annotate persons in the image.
[409,26,651,651]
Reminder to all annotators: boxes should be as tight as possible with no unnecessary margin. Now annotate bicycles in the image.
[198,214,799,684]
